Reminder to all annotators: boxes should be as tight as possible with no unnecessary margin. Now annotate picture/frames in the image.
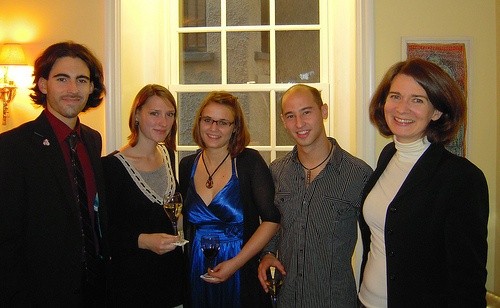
[402,38,471,157]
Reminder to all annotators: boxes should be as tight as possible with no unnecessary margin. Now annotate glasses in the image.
[199,116,236,129]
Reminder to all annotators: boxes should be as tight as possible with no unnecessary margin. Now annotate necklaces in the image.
[297,139,333,184]
[202,150,230,189]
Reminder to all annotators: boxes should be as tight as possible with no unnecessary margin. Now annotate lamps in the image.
[0,42,29,126]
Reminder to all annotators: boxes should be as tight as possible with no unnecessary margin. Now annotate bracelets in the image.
[262,252,275,258]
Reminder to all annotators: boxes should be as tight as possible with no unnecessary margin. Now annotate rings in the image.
[221,280,224,283]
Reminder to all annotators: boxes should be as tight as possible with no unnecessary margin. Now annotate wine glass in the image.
[264,270,283,308]
[200,234,220,279]
[164,192,183,236]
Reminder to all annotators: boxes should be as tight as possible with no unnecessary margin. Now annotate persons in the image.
[96,84,185,308]
[258,84,372,308]
[356,59,490,308]
[179,91,282,308]
[0,40,106,308]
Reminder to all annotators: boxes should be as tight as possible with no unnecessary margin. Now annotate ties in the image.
[66,131,97,287]
